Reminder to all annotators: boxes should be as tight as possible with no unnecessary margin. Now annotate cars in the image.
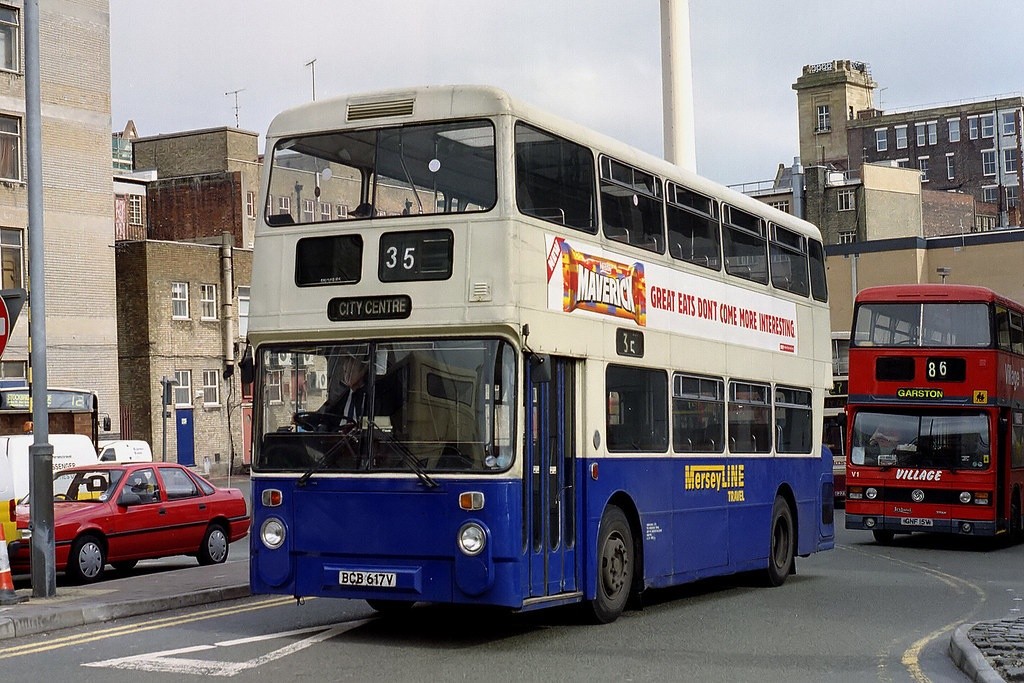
[7,461,250,586]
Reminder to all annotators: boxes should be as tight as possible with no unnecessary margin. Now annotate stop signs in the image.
[0,294,10,360]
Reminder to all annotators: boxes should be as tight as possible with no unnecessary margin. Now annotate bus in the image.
[0,387,112,457]
[837,284,1024,549]
[236,84,835,627]
[829,331,878,509]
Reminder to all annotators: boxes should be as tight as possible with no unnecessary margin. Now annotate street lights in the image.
[936,267,951,284]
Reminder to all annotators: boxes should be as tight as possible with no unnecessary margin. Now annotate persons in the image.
[126,473,155,500]
[347,203,377,217]
[870,416,907,457]
[290,359,388,434]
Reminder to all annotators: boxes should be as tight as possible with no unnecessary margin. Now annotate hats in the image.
[348,203,378,217]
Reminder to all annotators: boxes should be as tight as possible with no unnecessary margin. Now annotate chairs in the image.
[87,475,107,491]
[128,473,152,501]
[649,424,783,452]
[520,207,793,291]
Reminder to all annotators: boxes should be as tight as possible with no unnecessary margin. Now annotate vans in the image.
[0,435,105,550]
[94,440,153,485]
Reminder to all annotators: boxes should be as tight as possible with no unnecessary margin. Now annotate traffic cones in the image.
[0,523,29,606]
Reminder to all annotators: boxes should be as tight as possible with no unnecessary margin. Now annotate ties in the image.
[338,389,355,431]
